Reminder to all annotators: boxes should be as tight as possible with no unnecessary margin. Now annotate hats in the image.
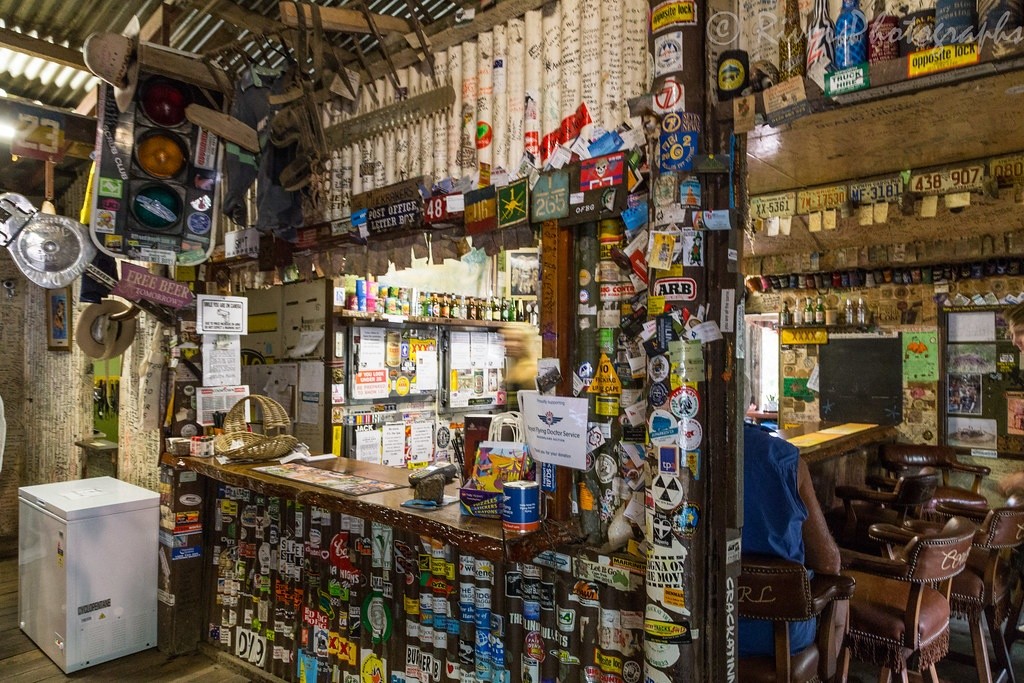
[74,300,137,360]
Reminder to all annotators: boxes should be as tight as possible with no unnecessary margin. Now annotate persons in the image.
[958,391,975,413]
[1008,299,1024,354]
[738,373,847,659]
[502,321,541,410]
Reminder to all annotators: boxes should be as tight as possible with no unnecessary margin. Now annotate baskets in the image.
[215,395,300,462]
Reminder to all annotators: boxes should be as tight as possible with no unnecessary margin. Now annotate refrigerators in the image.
[16,475,160,675]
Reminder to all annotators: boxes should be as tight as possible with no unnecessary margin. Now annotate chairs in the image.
[734,442,1024,683]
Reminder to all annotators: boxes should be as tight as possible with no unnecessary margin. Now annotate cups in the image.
[502,481,542,535]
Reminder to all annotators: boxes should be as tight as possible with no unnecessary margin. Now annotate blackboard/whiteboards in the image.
[817,332,903,424]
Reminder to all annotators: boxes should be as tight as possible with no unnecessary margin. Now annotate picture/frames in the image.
[45,284,72,352]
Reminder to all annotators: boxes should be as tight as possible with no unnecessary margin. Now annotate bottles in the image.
[779,295,870,327]
[416,291,542,326]
[779,0,1024,84]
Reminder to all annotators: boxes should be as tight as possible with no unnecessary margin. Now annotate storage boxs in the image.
[459,475,504,518]
[224,228,259,259]
